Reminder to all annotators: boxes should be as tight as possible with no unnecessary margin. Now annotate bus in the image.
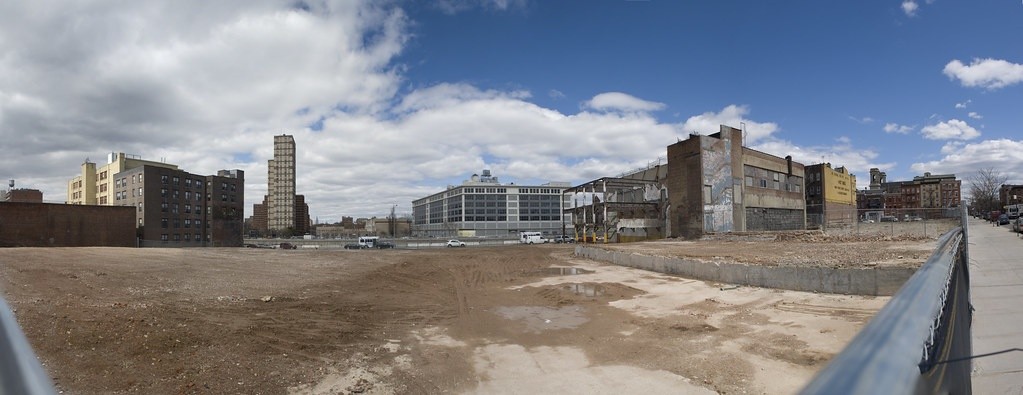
[519,231,541,245]
[358,236,380,248]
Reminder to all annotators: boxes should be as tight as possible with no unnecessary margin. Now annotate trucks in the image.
[526,235,547,245]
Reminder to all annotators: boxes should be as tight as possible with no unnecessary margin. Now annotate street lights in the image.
[1013,194,1019,237]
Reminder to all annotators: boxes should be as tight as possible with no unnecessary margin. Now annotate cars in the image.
[1012,216,1023,233]
[246,242,297,250]
[344,242,365,251]
[990,210,1002,222]
[374,240,396,250]
[997,214,1010,225]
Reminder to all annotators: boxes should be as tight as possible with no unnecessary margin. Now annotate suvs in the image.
[445,239,466,248]
[554,234,575,244]
